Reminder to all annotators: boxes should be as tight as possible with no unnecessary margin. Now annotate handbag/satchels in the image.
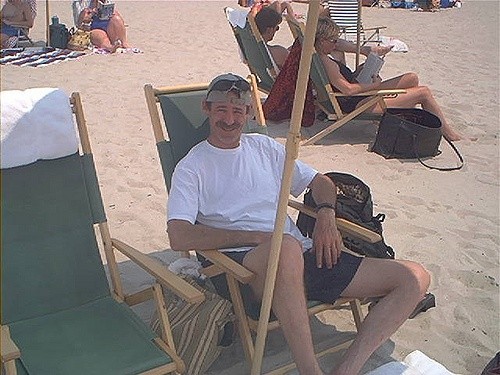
[371,107,463,170]
[153,273,235,375]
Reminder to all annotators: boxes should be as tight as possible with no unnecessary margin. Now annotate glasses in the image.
[326,38,337,43]
[206,79,250,99]
[268,26,279,31]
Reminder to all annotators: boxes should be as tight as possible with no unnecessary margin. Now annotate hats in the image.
[206,73,252,106]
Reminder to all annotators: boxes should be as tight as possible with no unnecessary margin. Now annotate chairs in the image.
[144,75,386,375]
[0,88,206,374]
[224,0,406,149]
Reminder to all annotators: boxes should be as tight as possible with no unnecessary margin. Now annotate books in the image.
[96,1,115,19]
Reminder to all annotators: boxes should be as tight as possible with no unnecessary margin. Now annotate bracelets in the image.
[314,203,336,210]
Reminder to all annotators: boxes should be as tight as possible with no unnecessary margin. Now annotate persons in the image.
[254,7,395,71]
[167,73,430,375]
[79,0,130,54]
[314,16,479,141]
[0,0,33,46]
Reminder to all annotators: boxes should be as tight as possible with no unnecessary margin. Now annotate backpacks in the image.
[67,29,90,51]
[297,172,395,259]
